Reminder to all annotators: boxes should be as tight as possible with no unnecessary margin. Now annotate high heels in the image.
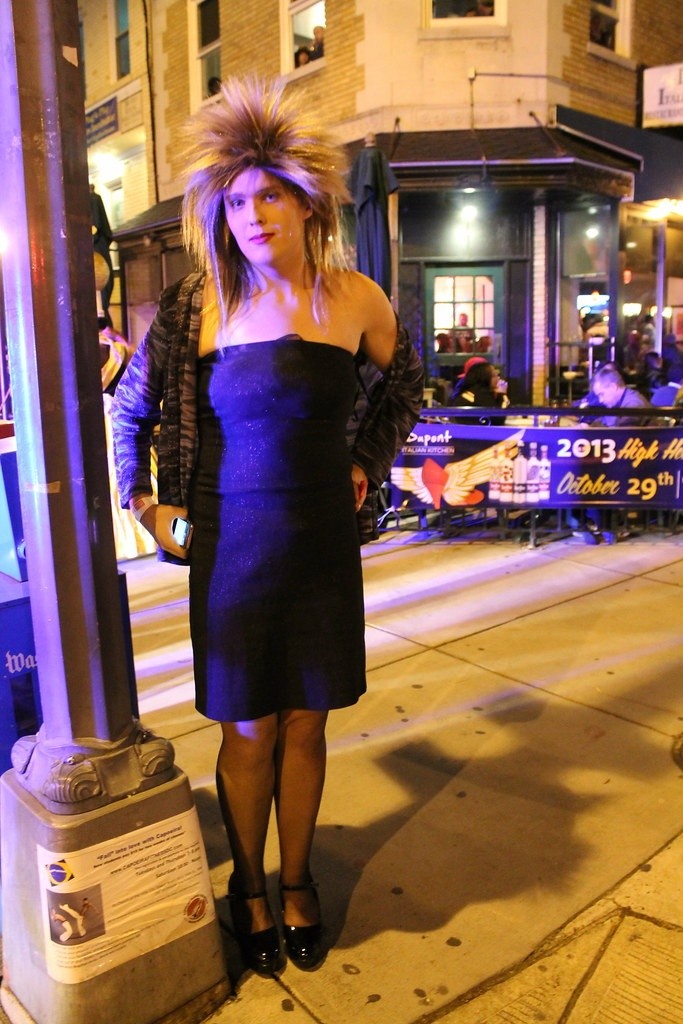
[278,869,326,970]
[224,869,283,975]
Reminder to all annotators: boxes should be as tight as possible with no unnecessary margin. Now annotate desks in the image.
[506,413,579,428]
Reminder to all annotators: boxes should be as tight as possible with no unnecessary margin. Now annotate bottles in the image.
[498,448,514,503]
[512,439,528,504]
[526,442,540,503]
[488,445,500,499]
[540,445,551,500]
[548,399,559,427]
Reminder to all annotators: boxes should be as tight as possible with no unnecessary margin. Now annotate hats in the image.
[457,356,487,377]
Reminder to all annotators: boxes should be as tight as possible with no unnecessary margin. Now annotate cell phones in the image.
[171,516,195,549]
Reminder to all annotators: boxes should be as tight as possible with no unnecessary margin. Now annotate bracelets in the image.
[130,497,153,523]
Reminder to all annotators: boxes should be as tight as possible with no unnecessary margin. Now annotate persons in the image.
[310,26,324,59]
[108,76,423,972]
[295,46,310,66]
[430,312,683,542]
[208,78,221,96]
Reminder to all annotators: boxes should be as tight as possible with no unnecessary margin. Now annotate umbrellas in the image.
[350,134,399,301]
